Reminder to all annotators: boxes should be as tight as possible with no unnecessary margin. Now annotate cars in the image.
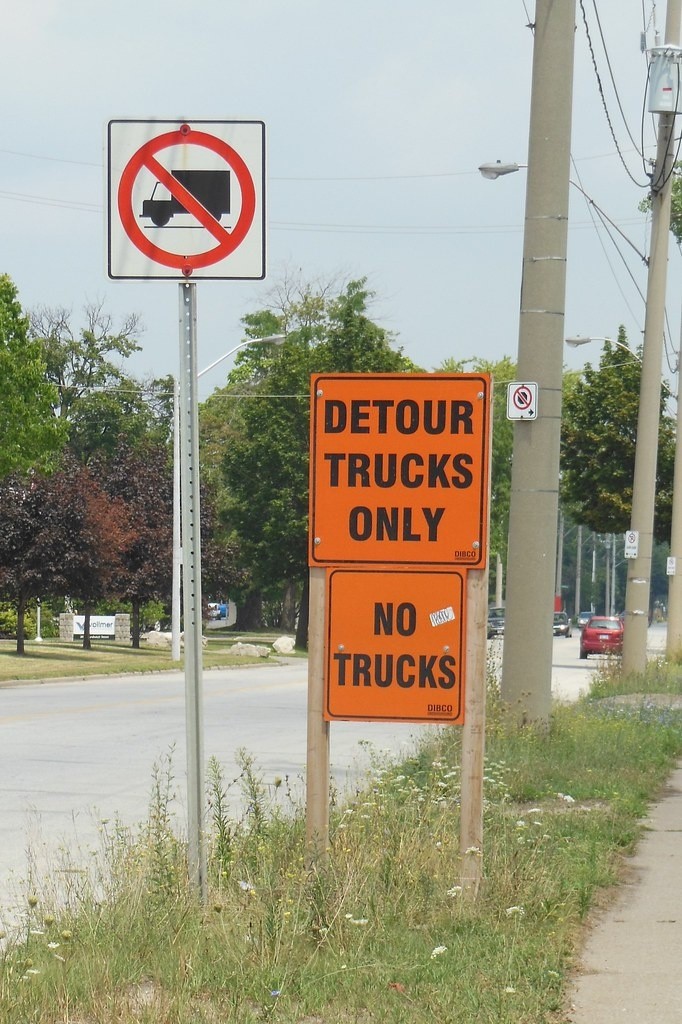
[211,604,229,618]
[577,612,597,628]
[616,610,652,628]
[486,607,505,639]
[580,616,624,659]
[552,612,572,638]
[208,601,221,609]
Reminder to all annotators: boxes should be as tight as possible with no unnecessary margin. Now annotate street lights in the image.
[172,334,287,660]
[480,163,682,664]
[565,337,678,402]
